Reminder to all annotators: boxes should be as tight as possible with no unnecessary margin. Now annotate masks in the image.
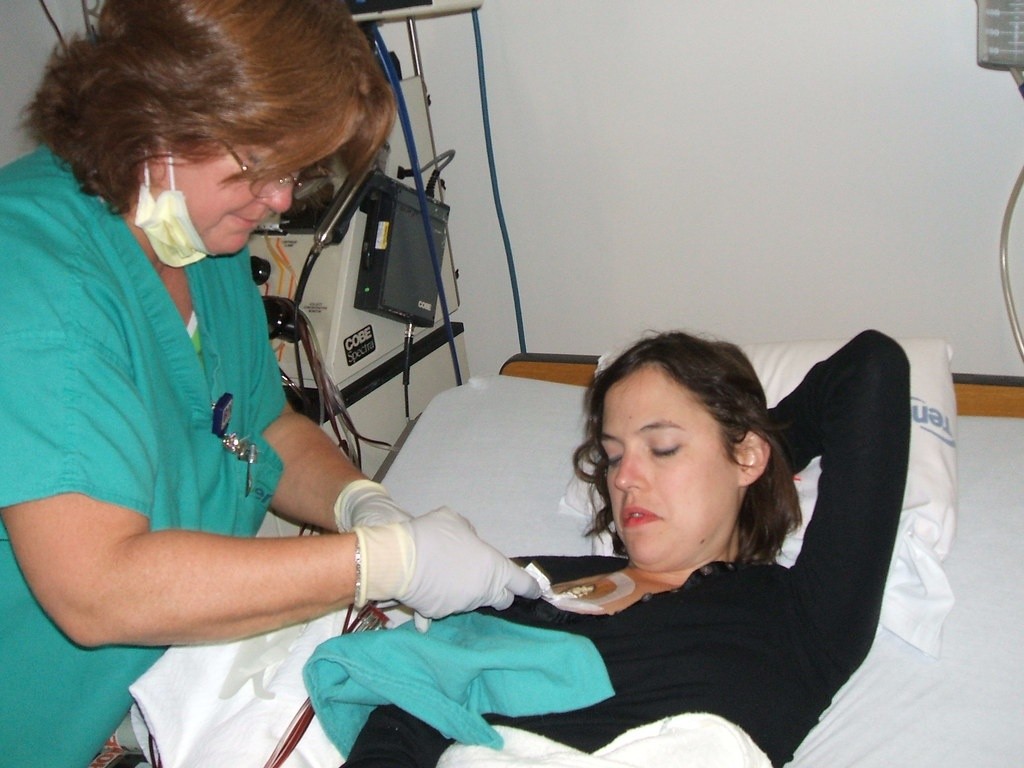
[134,148,215,268]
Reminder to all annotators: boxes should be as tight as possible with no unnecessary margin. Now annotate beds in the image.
[129,337,1024,768]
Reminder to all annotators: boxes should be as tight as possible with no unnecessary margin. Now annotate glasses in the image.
[221,138,333,201]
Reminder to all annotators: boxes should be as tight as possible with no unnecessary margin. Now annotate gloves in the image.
[333,478,432,634]
[354,505,542,618]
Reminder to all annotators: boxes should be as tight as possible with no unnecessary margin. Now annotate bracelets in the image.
[355,540,361,604]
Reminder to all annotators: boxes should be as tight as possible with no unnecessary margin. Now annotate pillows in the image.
[565,337,957,659]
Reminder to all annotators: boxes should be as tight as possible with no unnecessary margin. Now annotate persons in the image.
[339,329,912,768]
[0,0,537,768]
